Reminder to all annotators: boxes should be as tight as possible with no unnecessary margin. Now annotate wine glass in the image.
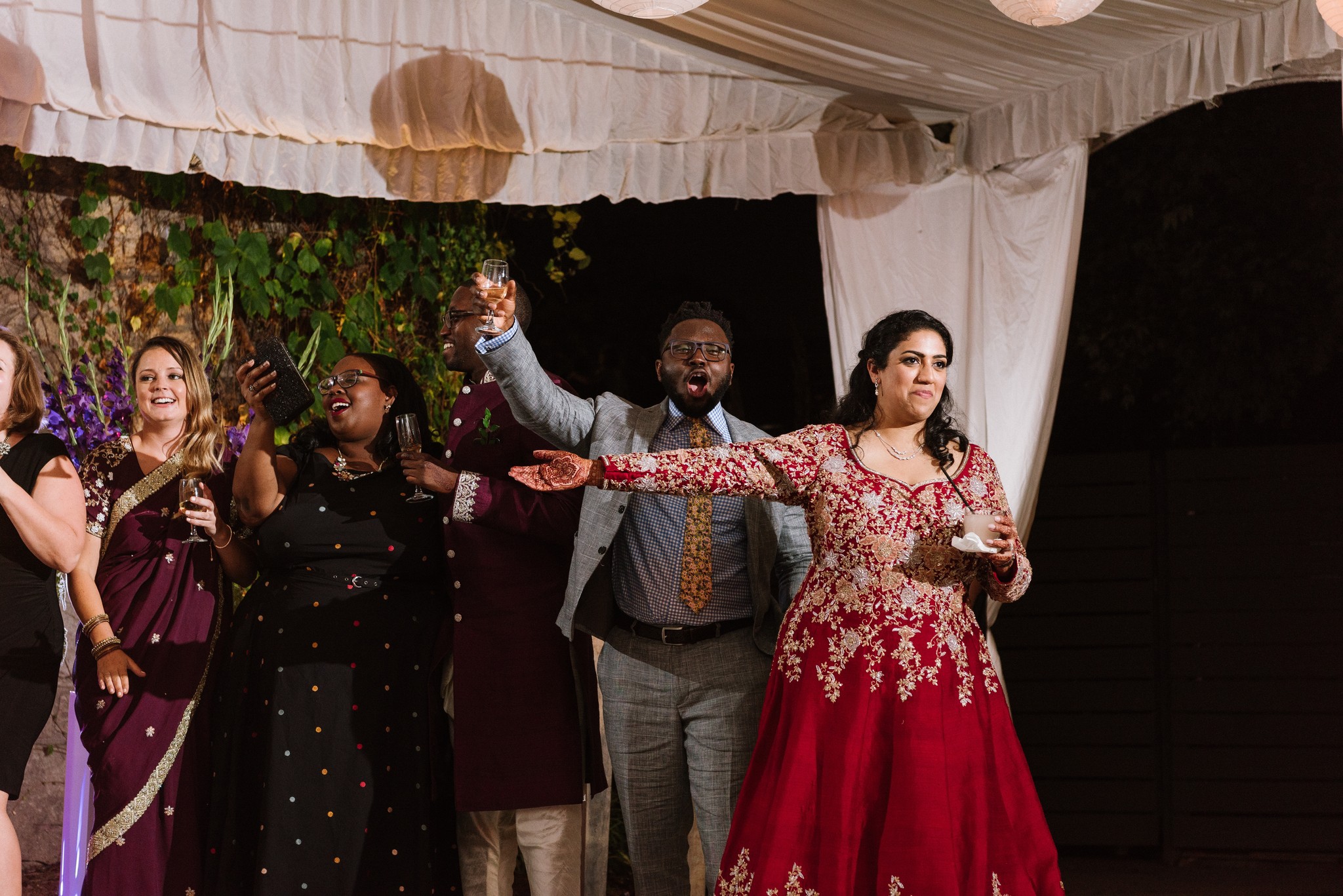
[475,258,509,336]
[396,413,434,502]
[178,479,210,543]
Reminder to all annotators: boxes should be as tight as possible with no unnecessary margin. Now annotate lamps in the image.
[591,0,711,20]
[1315,0,1343,38]
[990,1,1104,28]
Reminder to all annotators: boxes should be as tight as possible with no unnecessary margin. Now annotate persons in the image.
[472,271,812,895]
[399,279,605,896]
[0,327,87,896]
[232,355,444,896]
[508,309,1063,894]
[66,337,257,896]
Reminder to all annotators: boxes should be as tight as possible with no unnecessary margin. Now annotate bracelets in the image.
[91,636,121,661]
[214,523,232,549]
[82,613,109,638]
[991,548,1015,568]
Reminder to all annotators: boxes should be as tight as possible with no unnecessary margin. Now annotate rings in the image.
[248,385,257,394]
[103,672,111,677]
[1004,540,1014,552]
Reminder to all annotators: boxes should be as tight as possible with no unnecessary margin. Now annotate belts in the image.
[608,599,754,647]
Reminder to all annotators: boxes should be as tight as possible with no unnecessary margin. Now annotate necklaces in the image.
[334,444,388,481]
[869,417,925,460]
[0,430,12,456]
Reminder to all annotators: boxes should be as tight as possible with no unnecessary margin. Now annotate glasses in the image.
[441,310,475,329]
[661,339,732,362]
[316,369,388,396]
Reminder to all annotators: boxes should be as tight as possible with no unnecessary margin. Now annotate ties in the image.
[677,416,713,614]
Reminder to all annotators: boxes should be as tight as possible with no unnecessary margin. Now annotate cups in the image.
[961,507,1004,550]
